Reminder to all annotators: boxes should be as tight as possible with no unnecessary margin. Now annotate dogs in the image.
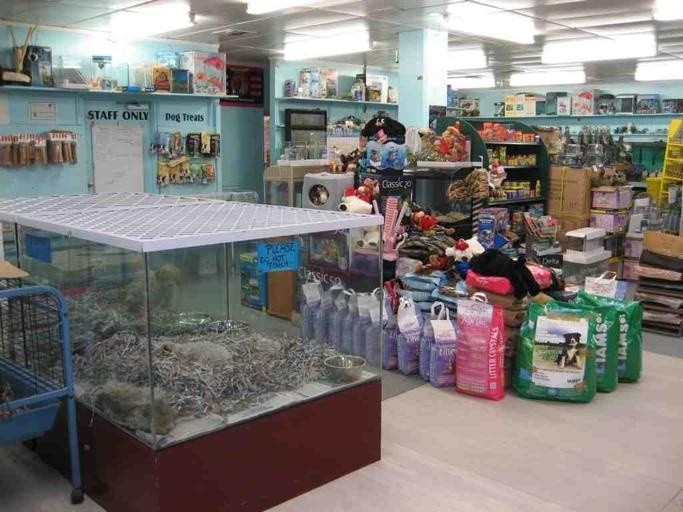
[552,332,582,368]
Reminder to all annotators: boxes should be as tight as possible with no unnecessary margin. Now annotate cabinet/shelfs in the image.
[435,114,551,218]
[276,96,400,130]
[454,110,679,153]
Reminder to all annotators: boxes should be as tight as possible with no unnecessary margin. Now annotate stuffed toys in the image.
[338,118,540,299]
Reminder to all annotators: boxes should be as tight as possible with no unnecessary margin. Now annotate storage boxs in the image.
[546,164,683,286]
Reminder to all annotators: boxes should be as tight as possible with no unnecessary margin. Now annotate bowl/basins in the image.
[325,355,365,381]
[177,312,211,329]
[209,319,249,333]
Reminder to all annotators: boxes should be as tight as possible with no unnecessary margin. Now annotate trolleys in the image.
[0,257,85,505]
